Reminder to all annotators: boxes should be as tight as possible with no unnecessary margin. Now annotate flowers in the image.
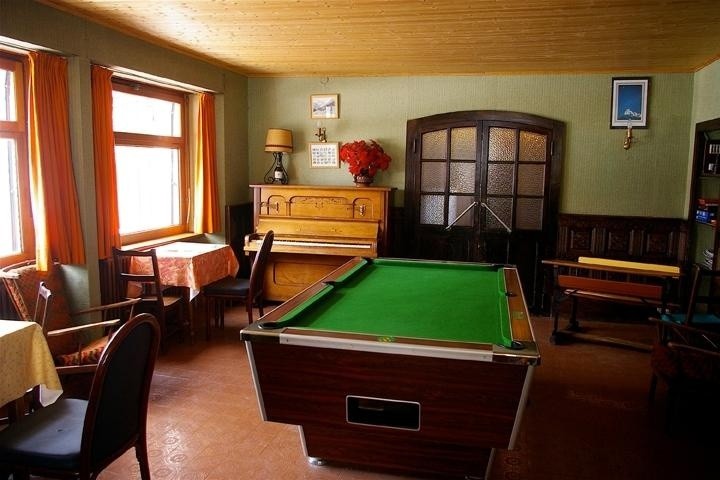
[339,138,391,179]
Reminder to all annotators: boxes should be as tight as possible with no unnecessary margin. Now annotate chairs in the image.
[0,310,162,480]
[0,280,56,426]
[200,227,276,343]
[643,314,720,410]
[658,263,720,349]
[109,243,188,357]
[1,256,143,378]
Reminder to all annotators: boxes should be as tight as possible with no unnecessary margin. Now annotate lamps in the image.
[262,127,294,185]
[621,116,634,150]
[311,118,329,144]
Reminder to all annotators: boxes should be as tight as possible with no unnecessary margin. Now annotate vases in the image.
[351,170,373,188]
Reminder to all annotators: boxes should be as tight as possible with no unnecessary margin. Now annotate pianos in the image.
[244,185,396,302]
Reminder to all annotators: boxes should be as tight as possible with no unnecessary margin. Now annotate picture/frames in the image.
[308,93,340,120]
[609,76,651,130]
[307,141,342,169]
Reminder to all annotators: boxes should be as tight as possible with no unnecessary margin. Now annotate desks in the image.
[121,239,241,348]
[232,251,547,480]
[0,319,67,480]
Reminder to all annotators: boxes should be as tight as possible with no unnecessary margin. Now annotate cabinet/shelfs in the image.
[679,117,720,312]
[537,251,686,356]
